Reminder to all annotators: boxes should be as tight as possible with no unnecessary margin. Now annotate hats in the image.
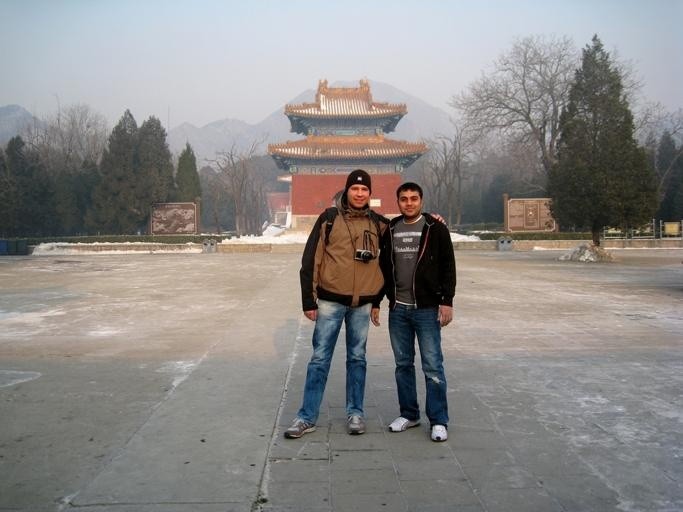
[345,170,371,193]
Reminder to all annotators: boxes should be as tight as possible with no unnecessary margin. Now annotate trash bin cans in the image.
[497,236,512,251]
[203,238,217,253]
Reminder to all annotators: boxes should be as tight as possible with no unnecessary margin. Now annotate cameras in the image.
[355,249,372,261]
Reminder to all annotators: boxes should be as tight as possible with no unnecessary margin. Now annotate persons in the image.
[369,182,456,443]
[284,169,447,438]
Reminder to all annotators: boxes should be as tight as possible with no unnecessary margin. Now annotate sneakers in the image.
[431,424,447,441]
[346,415,365,434]
[389,416,420,432]
[285,420,317,438]
